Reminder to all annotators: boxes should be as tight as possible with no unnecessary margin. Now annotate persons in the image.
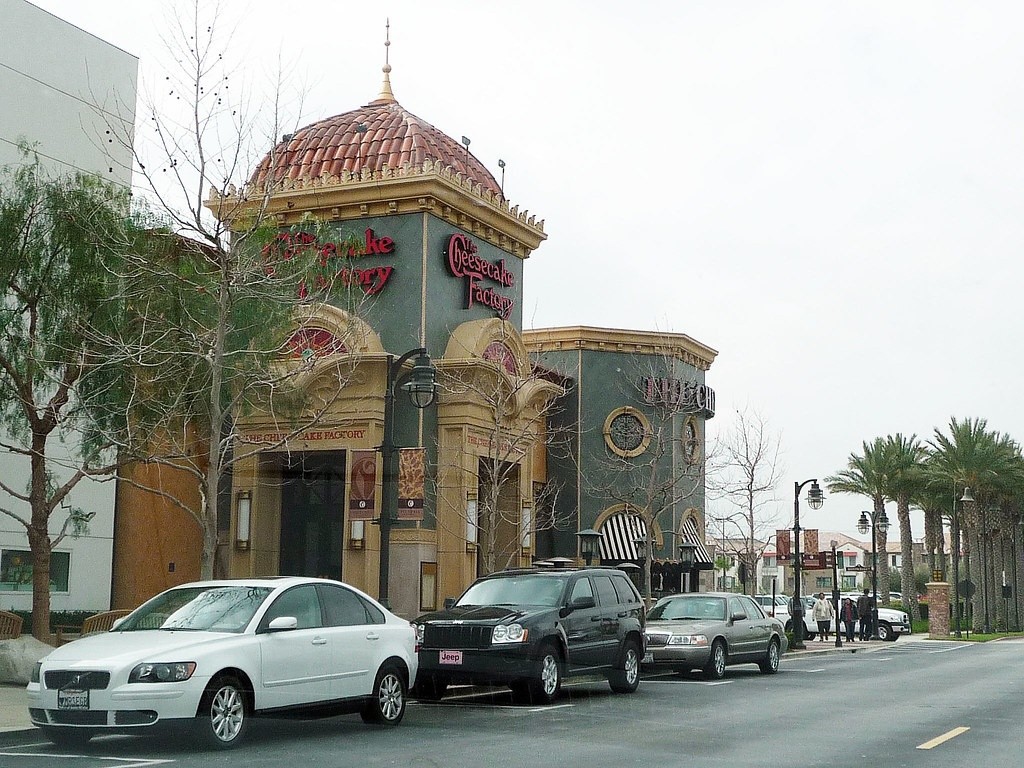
[787,592,806,620]
[857,589,874,641]
[812,592,834,642]
[840,598,859,642]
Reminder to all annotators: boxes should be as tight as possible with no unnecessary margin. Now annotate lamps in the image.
[462,136,471,179]
[523,500,530,556]
[236,490,250,551]
[932,566,942,582]
[355,125,367,182]
[350,521,364,550]
[282,133,294,172]
[498,159,506,198]
[467,493,477,554]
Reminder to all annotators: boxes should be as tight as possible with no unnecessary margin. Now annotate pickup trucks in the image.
[774,596,912,642]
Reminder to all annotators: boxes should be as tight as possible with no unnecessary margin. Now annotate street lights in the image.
[974,490,1004,636]
[789,478,827,649]
[951,478,977,639]
[715,518,730,592]
[856,508,892,640]
[376,347,437,610]
[1009,510,1024,636]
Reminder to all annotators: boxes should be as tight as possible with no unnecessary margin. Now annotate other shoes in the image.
[820,639,823,641]
[826,637,828,640]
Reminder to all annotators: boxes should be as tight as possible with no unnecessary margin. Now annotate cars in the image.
[24,576,421,749]
[637,590,789,679]
[745,584,913,628]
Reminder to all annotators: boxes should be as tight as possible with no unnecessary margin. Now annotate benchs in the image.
[0,610,171,647]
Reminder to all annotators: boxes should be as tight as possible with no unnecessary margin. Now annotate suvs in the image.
[408,562,647,705]
